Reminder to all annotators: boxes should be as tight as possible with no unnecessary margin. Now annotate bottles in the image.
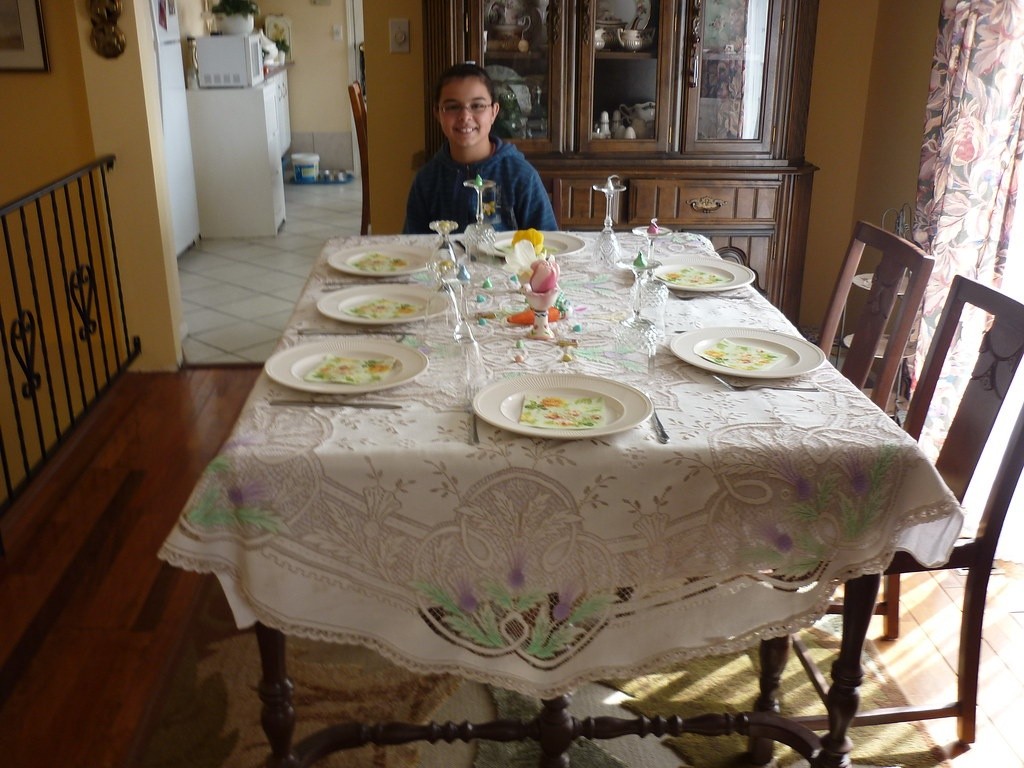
[595,28,605,51]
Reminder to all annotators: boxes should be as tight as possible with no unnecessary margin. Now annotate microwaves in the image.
[195,36,266,88]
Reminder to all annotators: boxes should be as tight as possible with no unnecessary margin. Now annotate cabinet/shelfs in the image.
[186,80,288,240]
[411,0,821,326]
[834,203,921,429]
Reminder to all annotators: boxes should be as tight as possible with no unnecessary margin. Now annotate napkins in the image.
[343,298,423,321]
[305,351,395,388]
[345,251,407,274]
[519,393,606,430]
[699,337,787,372]
[656,266,730,288]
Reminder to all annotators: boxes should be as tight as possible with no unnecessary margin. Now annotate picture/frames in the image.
[0,0,51,75]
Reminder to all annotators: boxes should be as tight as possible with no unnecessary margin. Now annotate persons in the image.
[402,63,563,233]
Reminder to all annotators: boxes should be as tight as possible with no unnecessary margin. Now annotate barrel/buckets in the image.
[291,153,320,181]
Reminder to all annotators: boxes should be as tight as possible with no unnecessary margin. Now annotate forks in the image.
[669,289,748,300]
[713,375,821,393]
[465,384,480,442]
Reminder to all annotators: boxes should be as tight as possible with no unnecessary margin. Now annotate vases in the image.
[524,291,560,340]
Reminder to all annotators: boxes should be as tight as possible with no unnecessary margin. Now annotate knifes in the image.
[297,328,416,336]
[270,399,405,408]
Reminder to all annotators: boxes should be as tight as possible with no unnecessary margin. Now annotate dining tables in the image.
[156,232,963,768]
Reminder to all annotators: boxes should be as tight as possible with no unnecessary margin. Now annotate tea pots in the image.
[595,10,642,49]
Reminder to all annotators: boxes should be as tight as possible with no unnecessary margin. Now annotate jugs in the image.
[617,27,656,52]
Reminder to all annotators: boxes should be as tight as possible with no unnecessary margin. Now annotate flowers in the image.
[504,227,561,294]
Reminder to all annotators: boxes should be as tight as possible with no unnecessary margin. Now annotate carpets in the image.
[120,569,951,768]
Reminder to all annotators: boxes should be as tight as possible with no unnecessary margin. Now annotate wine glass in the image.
[429,221,668,403]
[592,184,627,268]
[464,179,495,269]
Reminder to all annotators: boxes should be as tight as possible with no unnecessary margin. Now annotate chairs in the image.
[347,80,371,236]
[543,177,563,230]
[808,221,936,643]
[787,273,1024,749]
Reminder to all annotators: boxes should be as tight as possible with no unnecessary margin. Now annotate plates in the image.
[480,229,585,259]
[264,340,428,395]
[596,0,651,34]
[327,245,430,275]
[670,327,826,378]
[474,370,655,439]
[317,286,448,326]
[653,254,757,292]
[483,64,532,121]
[705,0,746,52]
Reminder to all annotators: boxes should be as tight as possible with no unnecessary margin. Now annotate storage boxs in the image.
[266,69,293,158]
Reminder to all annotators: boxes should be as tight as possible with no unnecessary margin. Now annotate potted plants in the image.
[211,0,260,35]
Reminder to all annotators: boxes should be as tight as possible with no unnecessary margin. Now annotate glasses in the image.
[436,101,493,114]
